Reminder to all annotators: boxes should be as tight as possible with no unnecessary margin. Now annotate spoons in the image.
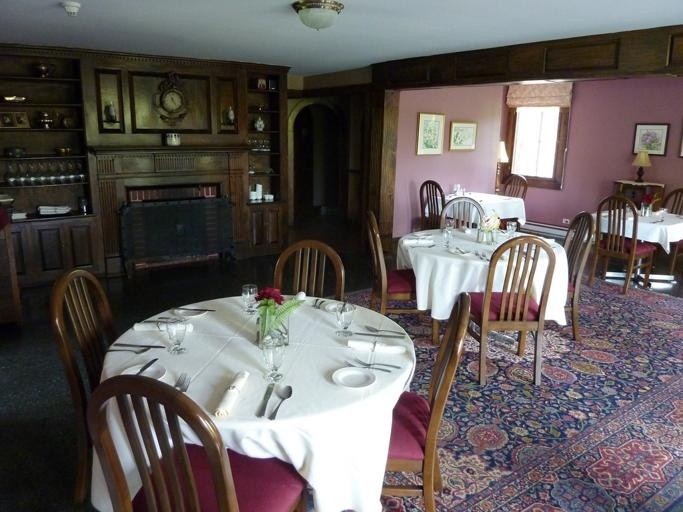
[270,385,293,420]
[365,324,407,336]
[107,347,151,355]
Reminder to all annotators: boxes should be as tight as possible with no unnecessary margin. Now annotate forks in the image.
[342,360,391,374]
[354,357,402,370]
[174,372,191,392]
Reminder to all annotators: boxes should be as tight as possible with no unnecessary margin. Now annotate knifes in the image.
[257,383,275,417]
[114,343,166,348]
[135,358,159,376]
[354,332,404,339]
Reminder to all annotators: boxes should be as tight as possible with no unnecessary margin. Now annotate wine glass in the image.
[241,284,259,314]
[336,302,355,338]
[165,320,187,356]
[263,342,285,384]
[440,219,517,251]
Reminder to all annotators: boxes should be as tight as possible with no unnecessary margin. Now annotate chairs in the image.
[417,180,446,226]
[272,237,344,306]
[52,268,123,459]
[380,293,472,511]
[465,236,555,386]
[441,197,486,236]
[86,375,308,511]
[561,210,595,342]
[659,188,682,276]
[592,194,655,294]
[365,211,439,346]
[504,175,529,202]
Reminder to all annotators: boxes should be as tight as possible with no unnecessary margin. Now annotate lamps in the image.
[291,1,345,32]
[631,151,653,183]
[496,140,509,195]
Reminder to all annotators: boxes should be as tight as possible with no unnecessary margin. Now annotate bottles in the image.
[227,105,237,125]
[108,101,117,120]
[78,197,87,215]
[4,160,88,184]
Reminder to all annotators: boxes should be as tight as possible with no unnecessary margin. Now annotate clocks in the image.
[151,71,191,124]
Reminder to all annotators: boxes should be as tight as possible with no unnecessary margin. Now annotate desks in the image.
[104,291,416,463]
[587,206,683,247]
[0,195,23,328]
[397,228,568,316]
[444,192,526,231]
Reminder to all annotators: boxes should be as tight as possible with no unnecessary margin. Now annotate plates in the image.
[119,363,167,380]
[332,367,376,389]
[411,232,472,256]
[174,305,210,318]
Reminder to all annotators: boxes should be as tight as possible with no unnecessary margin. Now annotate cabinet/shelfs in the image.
[1,52,107,286]
[245,71,289,258]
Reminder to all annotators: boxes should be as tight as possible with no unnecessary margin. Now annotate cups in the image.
[254,107,266,132]
[659,207,668,219]
[269,79,276,89]
[453,184,460,194]
[248,136,273,149]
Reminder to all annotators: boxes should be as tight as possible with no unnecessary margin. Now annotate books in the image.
[12,212,28,220]
[38,204,71,215]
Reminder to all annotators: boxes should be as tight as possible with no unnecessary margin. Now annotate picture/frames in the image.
[632,123,670,157]
[448,122,478,151]
[416,112,446,155]
